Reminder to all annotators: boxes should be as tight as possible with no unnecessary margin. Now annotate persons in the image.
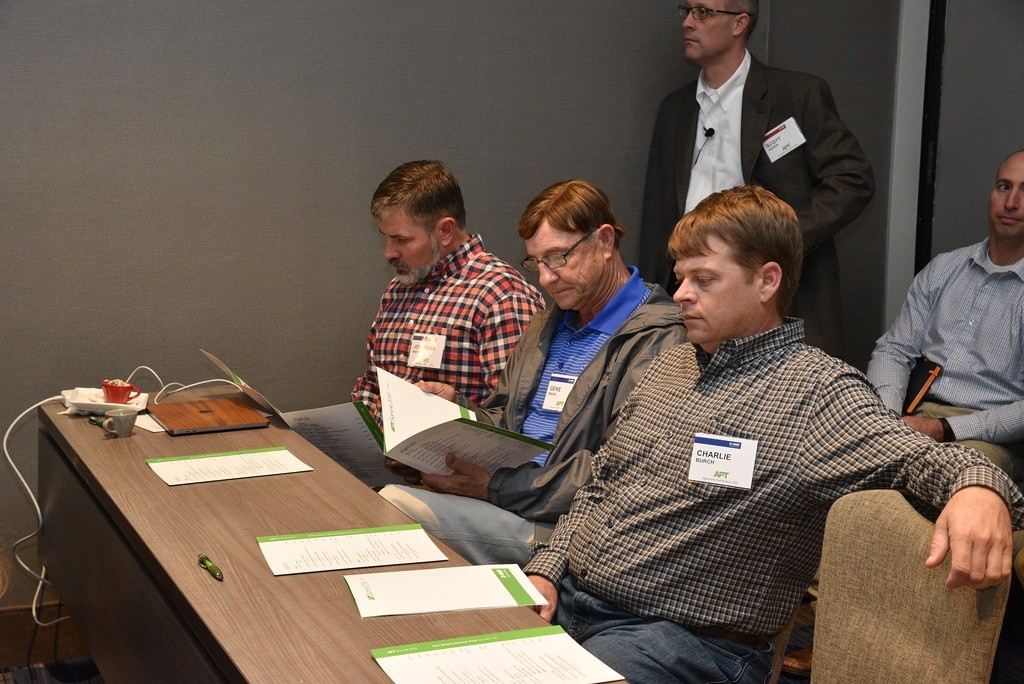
[640,0,875,359]
[350,159,546,483]
[379,179,688,564]
[522,186,1024,683]
[867,149,1024,482]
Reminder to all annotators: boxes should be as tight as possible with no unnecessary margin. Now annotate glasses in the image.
[522,229,595,274]
[678,5,755,21]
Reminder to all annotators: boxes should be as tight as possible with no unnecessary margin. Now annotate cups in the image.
[102,409,137,438]
[102,380,141,404]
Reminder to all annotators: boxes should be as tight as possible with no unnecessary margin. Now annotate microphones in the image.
[705,128,715,137]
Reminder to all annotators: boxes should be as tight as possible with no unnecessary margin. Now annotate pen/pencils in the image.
[197,553,224,580]
[89,416,103,427]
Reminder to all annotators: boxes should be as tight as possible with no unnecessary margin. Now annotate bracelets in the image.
[941,417,956,442]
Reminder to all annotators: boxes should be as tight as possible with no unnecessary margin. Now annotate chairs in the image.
[809,490,1010,682]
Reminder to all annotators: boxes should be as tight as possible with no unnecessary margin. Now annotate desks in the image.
[37,385,631,684]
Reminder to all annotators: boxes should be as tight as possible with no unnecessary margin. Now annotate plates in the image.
[69,388,149,413]
